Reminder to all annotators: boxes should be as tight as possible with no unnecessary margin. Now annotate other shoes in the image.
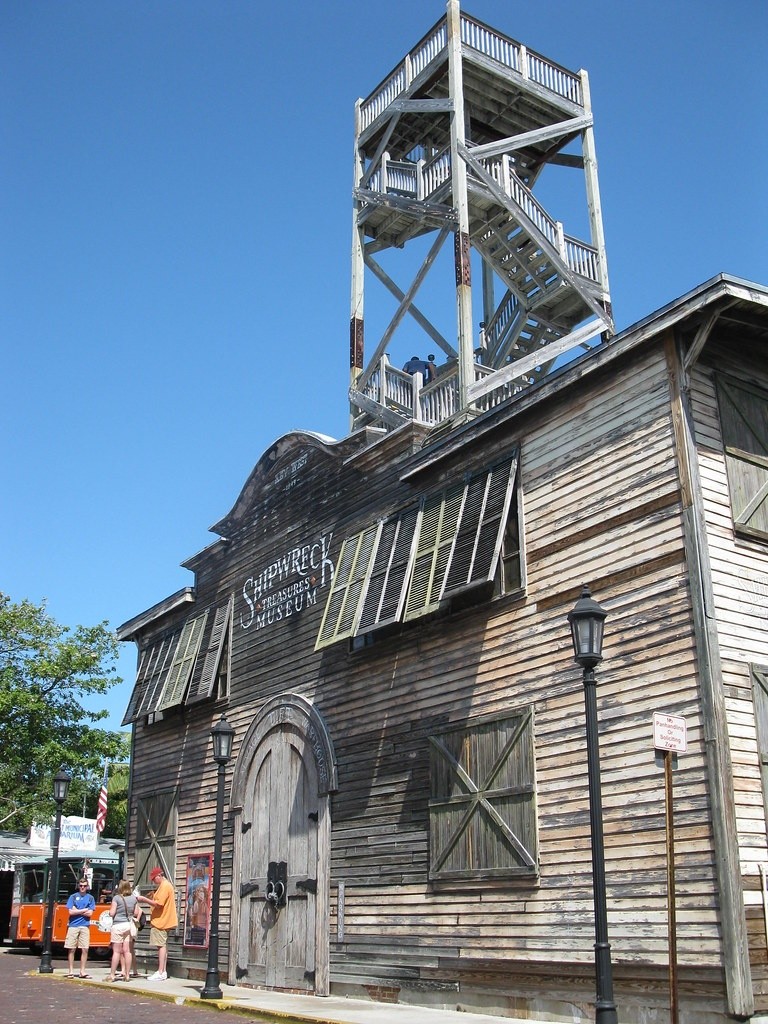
[131,973,138,978]
[118,973,125,977]
[147,971,167,981]
[103,976,115,982]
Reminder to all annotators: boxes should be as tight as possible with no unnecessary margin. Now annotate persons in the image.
[137,867,177,980]
[64,878,95,979]
[102,880,144,982]
[403,357,436,410]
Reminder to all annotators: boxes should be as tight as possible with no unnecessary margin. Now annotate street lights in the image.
[38,766,73,974]
[199,711,236,1000]
[567,584,620,1024]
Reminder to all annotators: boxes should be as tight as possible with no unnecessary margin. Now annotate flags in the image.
[96,759,108,833]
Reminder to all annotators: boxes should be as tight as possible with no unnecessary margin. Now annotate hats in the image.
[149,868,163,881]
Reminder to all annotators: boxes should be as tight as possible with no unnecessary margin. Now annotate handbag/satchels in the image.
[138,913,147,931]
[130,921,138,941]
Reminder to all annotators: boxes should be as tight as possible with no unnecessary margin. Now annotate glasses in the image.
[79,885,87,888]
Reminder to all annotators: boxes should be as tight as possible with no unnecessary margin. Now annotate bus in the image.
[16,850,121,962]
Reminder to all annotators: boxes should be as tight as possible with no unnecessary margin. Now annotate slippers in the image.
[67,972,74,980]
[80,973,87,978]
